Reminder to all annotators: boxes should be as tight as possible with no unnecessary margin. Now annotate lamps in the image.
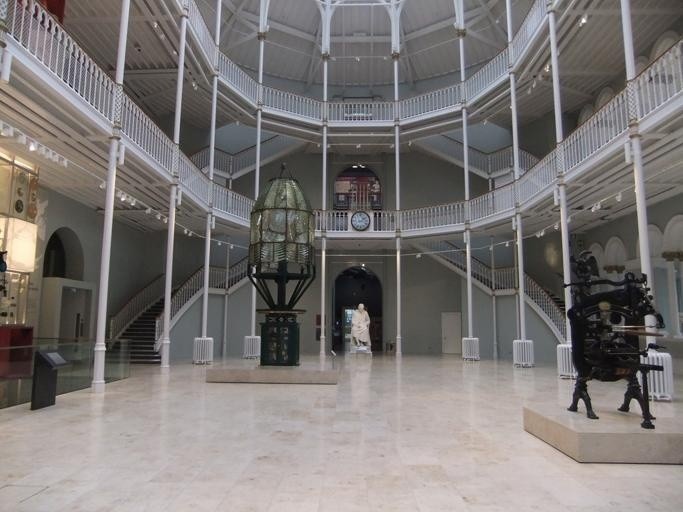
[323,51,392,65]
[0,116,683,265]
[148,14,595,150]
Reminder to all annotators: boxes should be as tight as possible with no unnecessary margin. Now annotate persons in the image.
[348,303,371,348]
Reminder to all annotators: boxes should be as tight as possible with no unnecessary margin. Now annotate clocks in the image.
[350,210,371,232]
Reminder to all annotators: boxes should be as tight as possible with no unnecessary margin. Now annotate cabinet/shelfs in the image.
[1,320,34,361]
